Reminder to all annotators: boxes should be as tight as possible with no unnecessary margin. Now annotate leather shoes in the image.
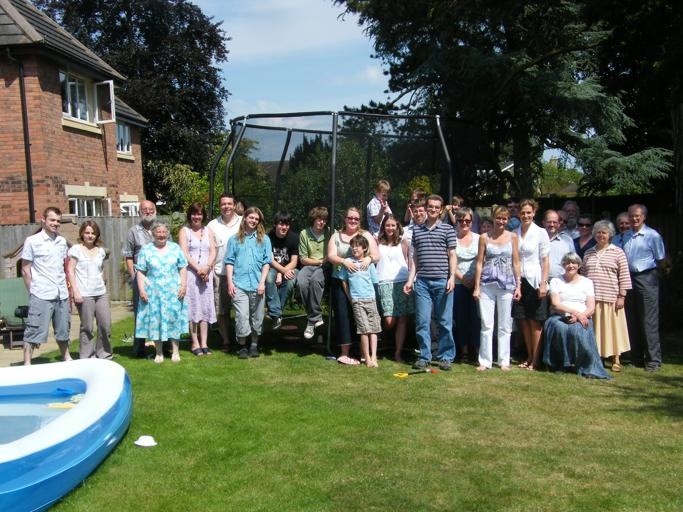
[272,316,283,330]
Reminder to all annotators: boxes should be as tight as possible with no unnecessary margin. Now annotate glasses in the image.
[578,222,592,227]
[457,219,472,224]
[346,217,360,221]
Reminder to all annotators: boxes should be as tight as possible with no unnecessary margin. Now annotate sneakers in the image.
[477,365,488,371]
[413,360,431,369]
[154,355,163,362]
[439,360,452,370]
[314,319,324,328]
[366,358,379,368]
[304,319,315,339]
[171,353,180,362]
[250,346,259,357]
[237,348,248,358]
[501,366,511,371]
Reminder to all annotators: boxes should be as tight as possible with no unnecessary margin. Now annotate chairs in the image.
[1,272,39,351]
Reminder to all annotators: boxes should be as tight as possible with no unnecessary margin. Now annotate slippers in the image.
[192,348,204,356]
[202,347,213,355]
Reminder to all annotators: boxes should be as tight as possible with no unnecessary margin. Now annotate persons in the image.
[123,179,665,379]
[65,221,114,359]
[21,206,73,366]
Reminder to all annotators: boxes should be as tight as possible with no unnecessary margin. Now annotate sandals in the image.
[612,363,623,372]
[518,361,538,370]
[223,344,232,353]
